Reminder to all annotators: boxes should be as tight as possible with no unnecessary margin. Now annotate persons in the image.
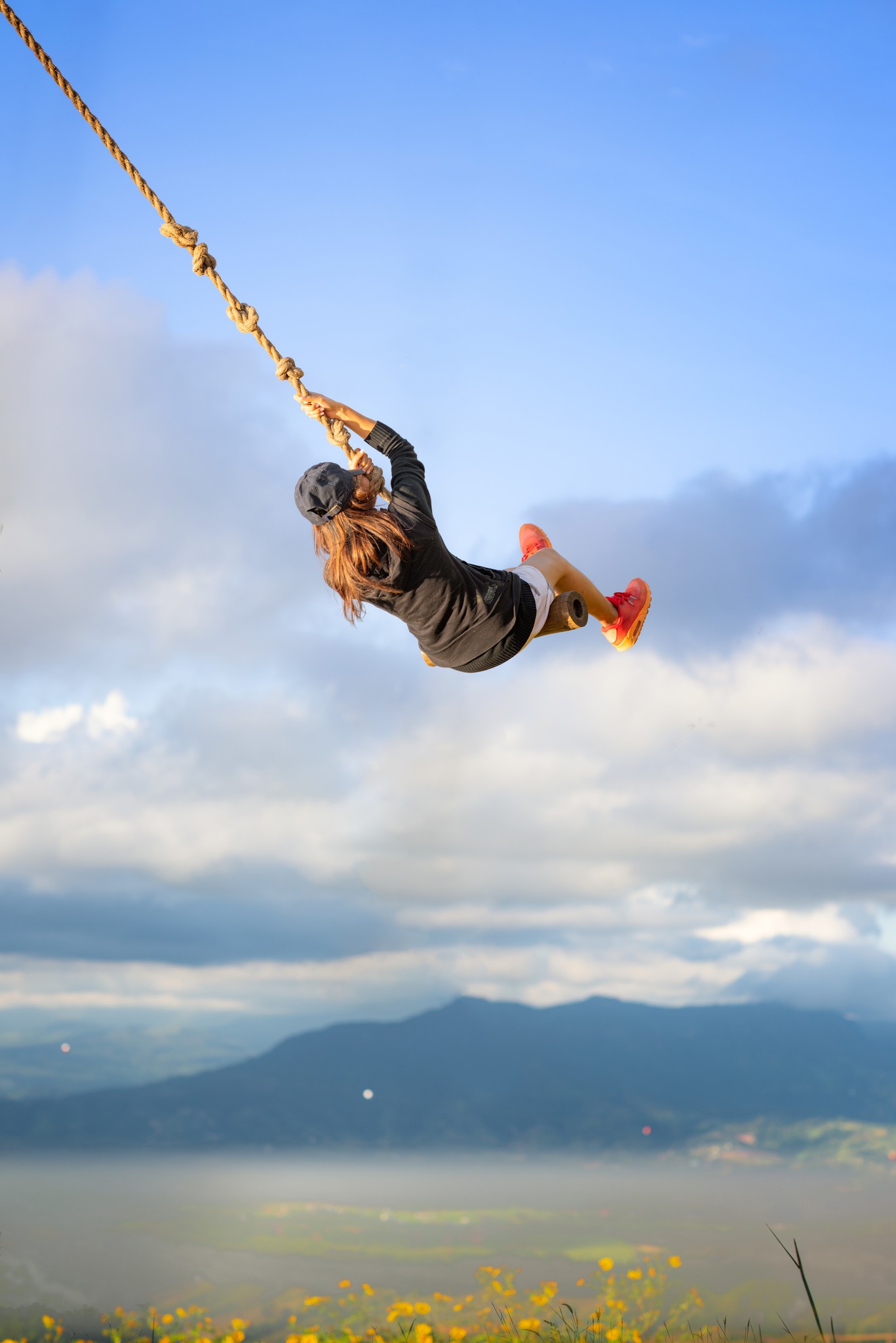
[295,390,651,673]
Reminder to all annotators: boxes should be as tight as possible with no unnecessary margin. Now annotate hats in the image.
[294,462,366,525]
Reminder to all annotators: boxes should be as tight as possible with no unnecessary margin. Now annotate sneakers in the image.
[600,577,651,652]
[519,523,552,564]
